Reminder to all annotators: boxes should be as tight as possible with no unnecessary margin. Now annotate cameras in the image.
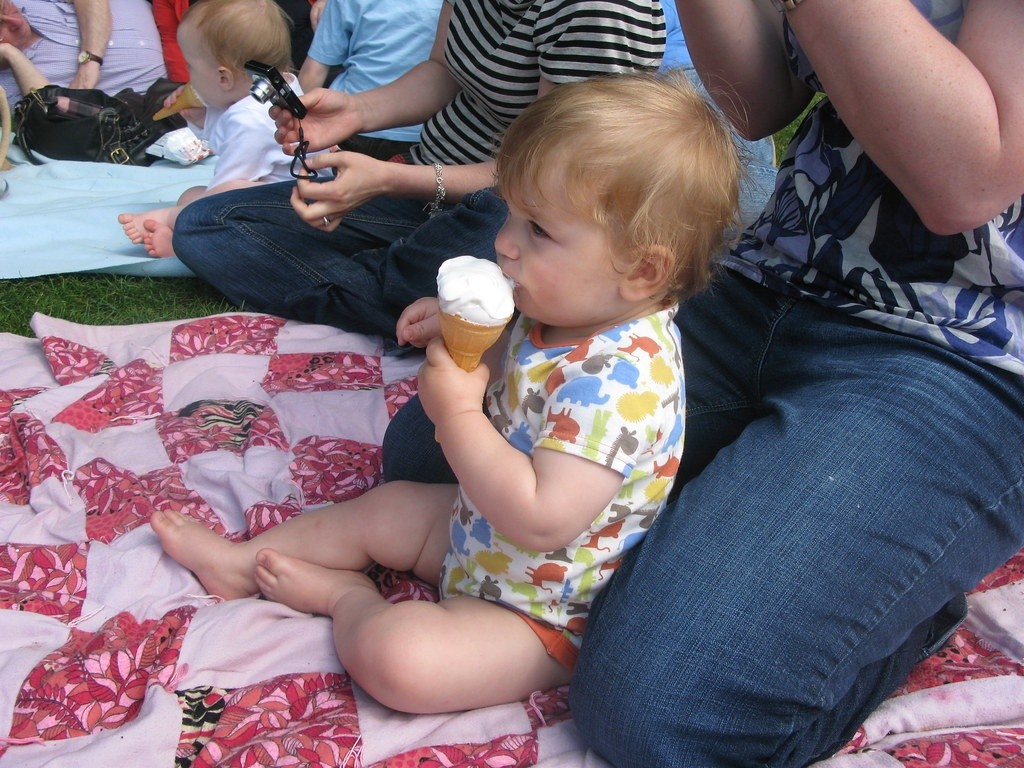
[243,60,307,120]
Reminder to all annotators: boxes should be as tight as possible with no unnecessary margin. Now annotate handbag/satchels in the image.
[13,78,187,167]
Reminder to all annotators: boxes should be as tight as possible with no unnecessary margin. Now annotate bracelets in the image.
[423,163,445,220]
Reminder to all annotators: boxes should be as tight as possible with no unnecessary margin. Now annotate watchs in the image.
[78,50,103,66]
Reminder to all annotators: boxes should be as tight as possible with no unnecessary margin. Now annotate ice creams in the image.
[153,84,207,121]
[436,255,515,443]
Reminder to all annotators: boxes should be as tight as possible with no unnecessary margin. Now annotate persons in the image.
[172,0,667,352]
[0,0,169,114]
[152,0,328,84]
[118,0,335,258]
[380,0,1024,768]
[298,0,444,163]
[150,74,739,715]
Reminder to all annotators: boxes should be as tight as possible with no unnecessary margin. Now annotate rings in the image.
[324,216,330,226]
